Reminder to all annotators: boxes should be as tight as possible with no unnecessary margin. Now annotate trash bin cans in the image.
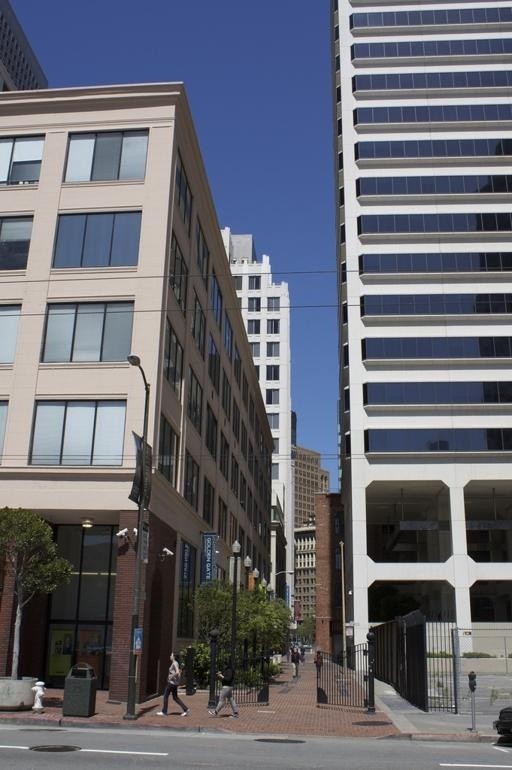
[63,662,97,717]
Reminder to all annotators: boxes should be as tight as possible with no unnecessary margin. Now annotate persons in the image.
[154,651,191,717]
[289,642,306,681]
[207,656,239,718]
[313,650,323,678]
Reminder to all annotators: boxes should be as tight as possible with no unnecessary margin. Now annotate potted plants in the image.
[2,503,75,711]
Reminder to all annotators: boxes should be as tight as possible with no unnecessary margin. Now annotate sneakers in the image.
[156,708,239,718]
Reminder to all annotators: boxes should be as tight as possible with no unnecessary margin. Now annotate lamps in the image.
[80,516,95,529]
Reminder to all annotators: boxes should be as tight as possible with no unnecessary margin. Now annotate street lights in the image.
[229,539,274,690]
[121,353,152,720]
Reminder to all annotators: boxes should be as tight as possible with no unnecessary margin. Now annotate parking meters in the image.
[468,671,479,733]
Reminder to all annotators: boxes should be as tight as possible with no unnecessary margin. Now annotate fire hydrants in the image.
[31,681,47,713]
